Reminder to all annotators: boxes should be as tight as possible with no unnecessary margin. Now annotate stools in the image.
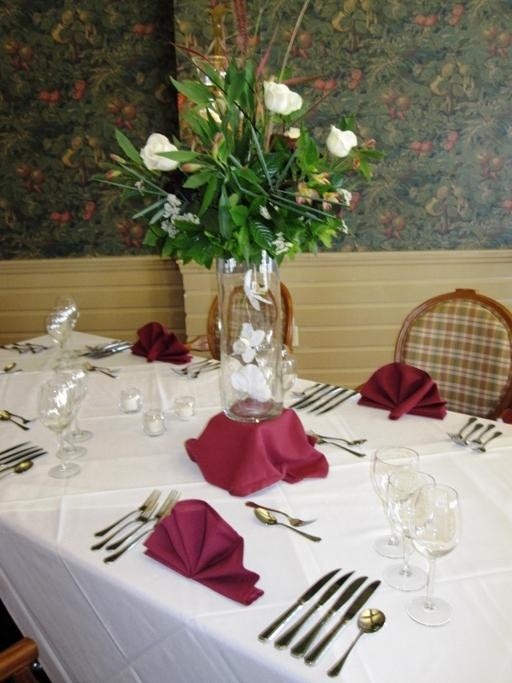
[0,622,47,679]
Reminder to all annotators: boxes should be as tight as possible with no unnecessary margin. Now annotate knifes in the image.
[258,566,382,665]
[1,437,50,474]
[288,385,357,415]
[81,338,132,358]
[0,368,24,373]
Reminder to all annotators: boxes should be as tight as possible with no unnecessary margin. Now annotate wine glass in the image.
[44,293,81,353]
[372,445,464,625]
[38,359,96,482]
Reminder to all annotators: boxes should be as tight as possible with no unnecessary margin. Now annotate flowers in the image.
[91,0,389,401]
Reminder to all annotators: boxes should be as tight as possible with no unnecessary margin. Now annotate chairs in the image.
[393,282,510,426]
[201,280,297,369]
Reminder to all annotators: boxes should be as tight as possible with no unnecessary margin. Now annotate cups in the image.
[173,395,197,421]
[119,386,144,414]
[144,409,168,437]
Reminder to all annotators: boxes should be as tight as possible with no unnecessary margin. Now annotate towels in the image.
[175,406,337,499]
[352,352,448,425]
[128,319,196,370]
[138,498,271,608]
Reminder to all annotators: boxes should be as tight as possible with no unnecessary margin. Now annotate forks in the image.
[246,499,317,526]
[93,485,183,564]
[4,409,37,424]
[170,356,223,383]
[309,429,365,459]
[0,340,52,353]
[85,360,122,379]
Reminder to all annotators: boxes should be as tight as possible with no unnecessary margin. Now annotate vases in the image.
[210,248,294,423]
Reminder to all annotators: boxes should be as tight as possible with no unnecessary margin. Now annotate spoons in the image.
[328,607,387,679]
[448,415,505,455]
[0,361,17,372]
[308,428,366,446]
[0,409,31,431]
[251,507,323,543]
